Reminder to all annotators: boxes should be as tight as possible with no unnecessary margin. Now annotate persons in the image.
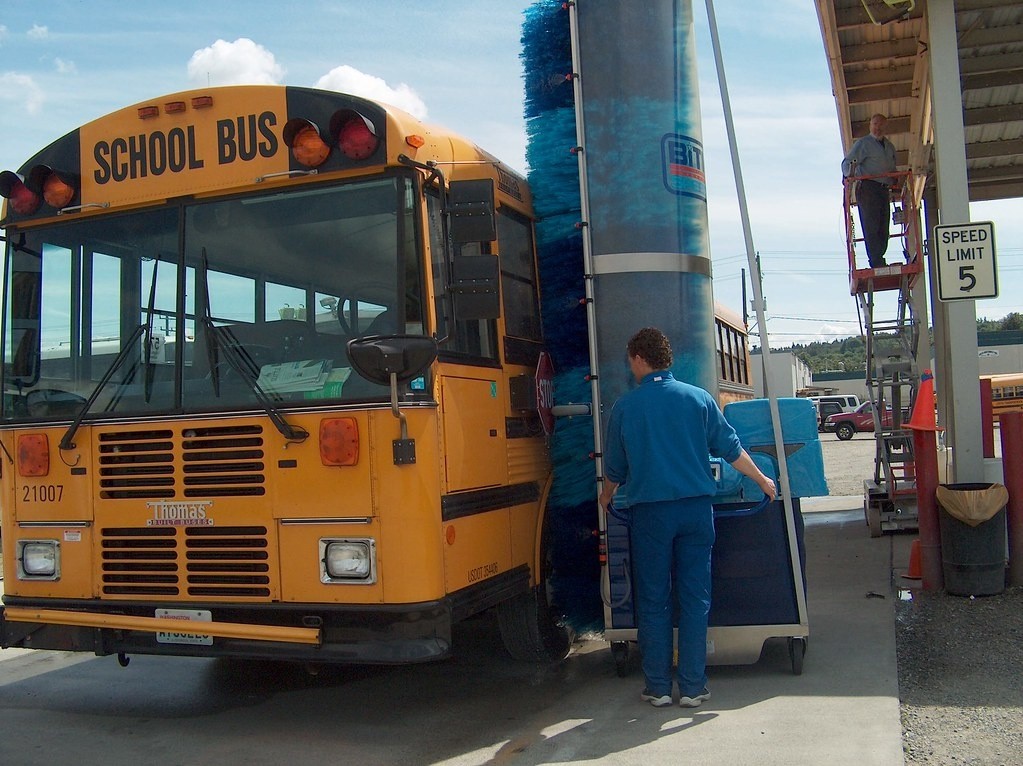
[600,326,777,706]
[841,113,901,269]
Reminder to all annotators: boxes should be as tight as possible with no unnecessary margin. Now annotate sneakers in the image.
[679,685,711,707]
[640,686,672,707]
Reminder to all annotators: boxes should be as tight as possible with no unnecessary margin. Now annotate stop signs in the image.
[532,346,557,433]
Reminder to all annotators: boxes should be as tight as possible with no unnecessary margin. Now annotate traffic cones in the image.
[900,369,945,432]
[901,538,922,580]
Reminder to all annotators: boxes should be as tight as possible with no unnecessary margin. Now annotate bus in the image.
[0,81,759,678]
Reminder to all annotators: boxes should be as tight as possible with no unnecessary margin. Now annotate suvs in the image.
[807,395,862,433]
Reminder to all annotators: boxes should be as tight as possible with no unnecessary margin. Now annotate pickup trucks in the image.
[823,399,912,440]
